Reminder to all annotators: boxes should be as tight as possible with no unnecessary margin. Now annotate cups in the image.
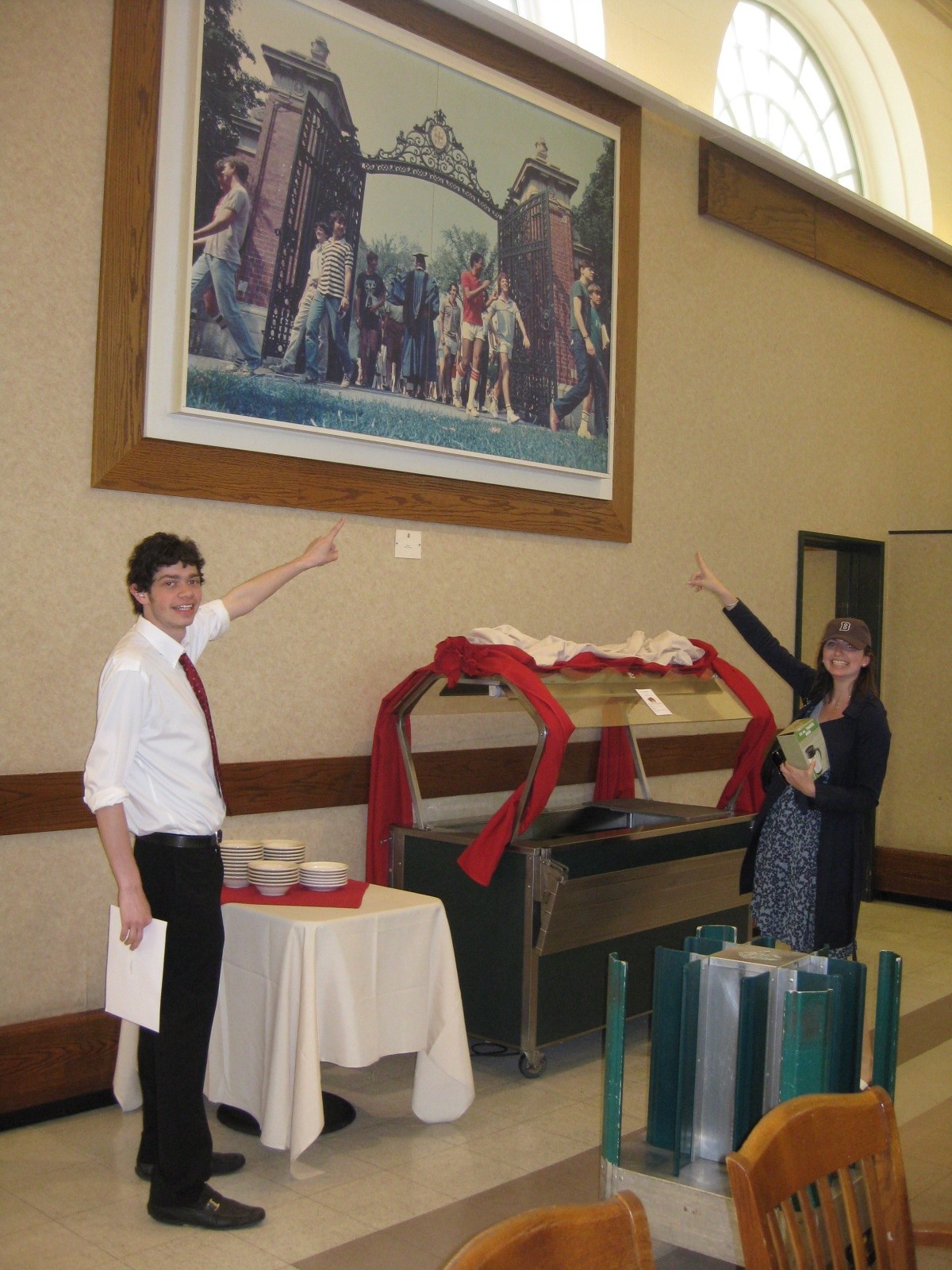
[805,744,823,774]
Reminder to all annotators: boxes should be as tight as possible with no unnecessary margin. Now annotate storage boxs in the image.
[775,717,832,783]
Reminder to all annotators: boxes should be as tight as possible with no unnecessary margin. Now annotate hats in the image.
[824,617,872,652]
[411,253,429,263]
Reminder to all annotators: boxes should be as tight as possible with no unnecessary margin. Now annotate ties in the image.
[212,652,221,799]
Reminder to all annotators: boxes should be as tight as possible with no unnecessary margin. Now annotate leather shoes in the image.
[134,1148,246,1182]
[147,1183,265,1230]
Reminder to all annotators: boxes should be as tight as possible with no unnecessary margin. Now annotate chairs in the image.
[724,1083,952,1270]
[440,1188,656,1270]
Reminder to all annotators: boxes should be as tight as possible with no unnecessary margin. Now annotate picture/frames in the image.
[89,0,644,544]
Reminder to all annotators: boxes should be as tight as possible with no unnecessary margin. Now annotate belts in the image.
[136,829,223,852]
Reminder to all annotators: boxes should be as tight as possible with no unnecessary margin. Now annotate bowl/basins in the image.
[220,839,304,894]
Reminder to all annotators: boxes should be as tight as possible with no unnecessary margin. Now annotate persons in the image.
[689,552,892,962]
[270,214,610,440]
[84,513,346,1230]
[194,157,259,374]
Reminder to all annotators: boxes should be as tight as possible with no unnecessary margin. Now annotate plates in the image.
[299,862,349,892]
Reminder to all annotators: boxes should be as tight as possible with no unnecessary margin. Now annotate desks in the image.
[111,876,447,1139]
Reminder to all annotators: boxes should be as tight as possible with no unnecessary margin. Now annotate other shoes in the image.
[577,429,595,440]
[293,374,318,387]
[224,355,262,371]
[340,366,354,389]
[452,381,462,408]
[549,403,560,433]
[466,404,479,417]
[507,414,520,424]
[268,364,296,377]
[489,404,499,418]
